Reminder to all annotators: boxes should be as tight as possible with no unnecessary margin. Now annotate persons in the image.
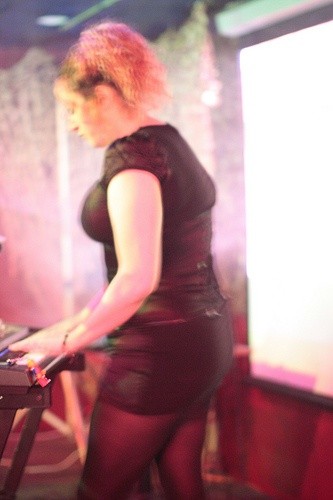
[7,22,234,500]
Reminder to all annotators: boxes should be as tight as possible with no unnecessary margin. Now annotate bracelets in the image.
[62,334,69,353]
